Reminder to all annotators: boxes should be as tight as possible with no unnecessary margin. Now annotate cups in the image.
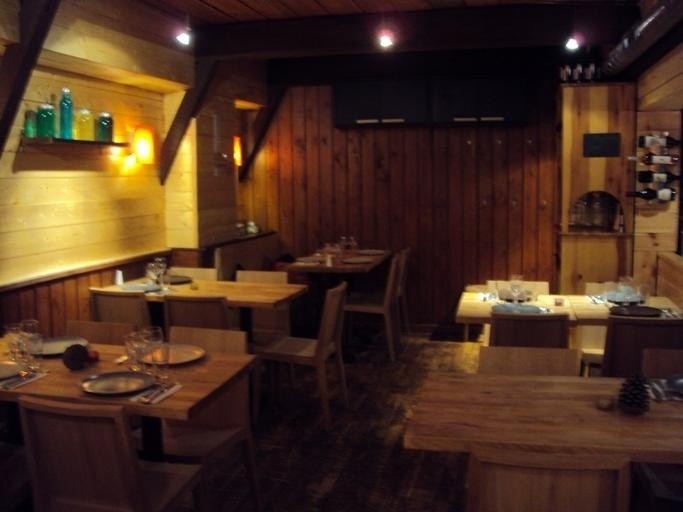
[152,343,169,377]
[26,334,42,368]
[126,331,144,370]
[4,323,24,361]
[153,343,169,379]
[147,263,159,286]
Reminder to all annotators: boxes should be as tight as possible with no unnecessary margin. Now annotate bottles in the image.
[93,107,113,143]
[637,169,681,184]
[627,152,680,166]
[22,109,39,140]
[36,98,57,139]
[76,107,96,142]
[637,134,682,148]
[56,86,74,138]
[624,187,677,202]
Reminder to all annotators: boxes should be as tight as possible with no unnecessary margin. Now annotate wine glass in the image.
[144,326,163,377]
[20,319,41,367]
[510,272,523,304]
[154,257,167,290]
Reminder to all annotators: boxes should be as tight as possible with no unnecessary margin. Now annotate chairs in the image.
[488,314,568,349]
[477,345,582,378]
[466,439,629,511]
[344,246,413,361]
[236,271,288,284]
[580,319,683,378]
[90,289,149,331]
[167,267,219,280]
[66,322,136,345]
[253,282,349,425]
[166,326,257,508]
[19,396,201,511]
[163,295,233,330]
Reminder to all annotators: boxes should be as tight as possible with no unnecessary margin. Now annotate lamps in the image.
[175,27,191,45]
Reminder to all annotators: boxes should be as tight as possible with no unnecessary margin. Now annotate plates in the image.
[0,361,20,380]
[168,275,192,284]
[602,292,644,302]
[357,250,384,255]
[609,306,662,317]
[31,335,89,356]
[119,284,160,292]
[80,371,156,396]
[298,257,326,262]
[139,342,206,365]
[341,257,373,263]
[491,304,539,315]
